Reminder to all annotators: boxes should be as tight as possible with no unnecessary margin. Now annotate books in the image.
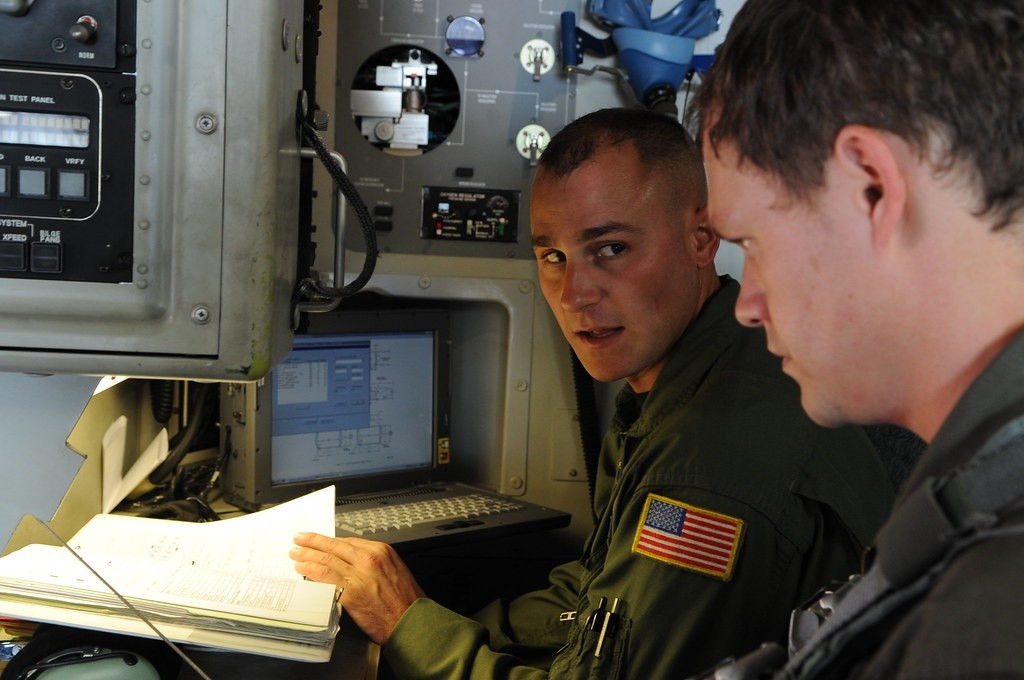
[0,482,343,663]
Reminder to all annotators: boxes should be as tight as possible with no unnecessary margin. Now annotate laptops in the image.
[248,310,572,551]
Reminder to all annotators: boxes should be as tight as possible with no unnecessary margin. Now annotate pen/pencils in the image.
[576,597,620,658]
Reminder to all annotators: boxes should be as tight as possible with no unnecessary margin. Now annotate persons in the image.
[287,107,918,680]
[674,2,1024,680]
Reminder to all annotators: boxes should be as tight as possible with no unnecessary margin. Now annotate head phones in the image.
[0,623,184,680]
